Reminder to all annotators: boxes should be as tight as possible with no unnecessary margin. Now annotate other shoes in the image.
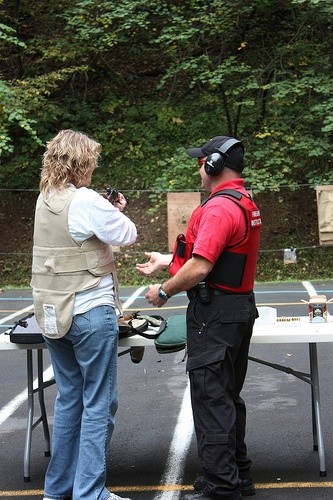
[181,490,240,500]
[194,476,257,495]
[106,492,131,500]
[43,495,55,500]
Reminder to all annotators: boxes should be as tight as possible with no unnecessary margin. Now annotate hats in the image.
[186,136,245,173]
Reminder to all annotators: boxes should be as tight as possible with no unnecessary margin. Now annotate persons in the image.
[31,129,137,500]
[135,136,261,500]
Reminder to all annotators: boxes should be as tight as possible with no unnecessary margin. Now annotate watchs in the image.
[158,285,168,301]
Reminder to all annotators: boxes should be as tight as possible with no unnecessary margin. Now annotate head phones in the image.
[205,139,244,176]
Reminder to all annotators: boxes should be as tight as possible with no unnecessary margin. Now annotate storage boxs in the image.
[301,294,333,322]
[255,306,277,326]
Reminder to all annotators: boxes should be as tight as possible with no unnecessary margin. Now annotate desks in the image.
[0,314,333,483]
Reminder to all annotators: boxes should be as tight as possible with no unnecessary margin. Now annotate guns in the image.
[116,310,141,331]
[99,187,129,205]
